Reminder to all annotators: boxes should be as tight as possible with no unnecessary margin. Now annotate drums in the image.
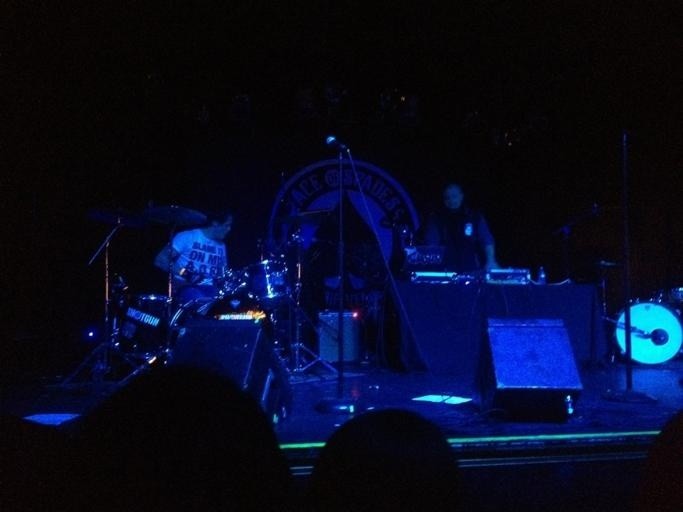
[247,259,292,304]
[220,311,270,333]
[134,292,169,320]
[612,292,683,367]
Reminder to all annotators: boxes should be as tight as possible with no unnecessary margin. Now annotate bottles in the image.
[539,267,546,284]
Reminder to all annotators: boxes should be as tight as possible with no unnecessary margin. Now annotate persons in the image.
[305,409,481,509]
[144,199,236,306]
[420,180,498,275]
[12,359,304,510]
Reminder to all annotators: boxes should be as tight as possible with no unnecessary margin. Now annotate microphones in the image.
[148,200,179,210]
[327,134,350,154]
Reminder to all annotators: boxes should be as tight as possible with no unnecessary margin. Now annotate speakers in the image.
[318,309,357,362]
[483,317,584,422]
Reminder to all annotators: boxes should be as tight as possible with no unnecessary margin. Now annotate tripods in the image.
[273,228,342,377]
[54,208,159,390]
[104,211,177,399]
